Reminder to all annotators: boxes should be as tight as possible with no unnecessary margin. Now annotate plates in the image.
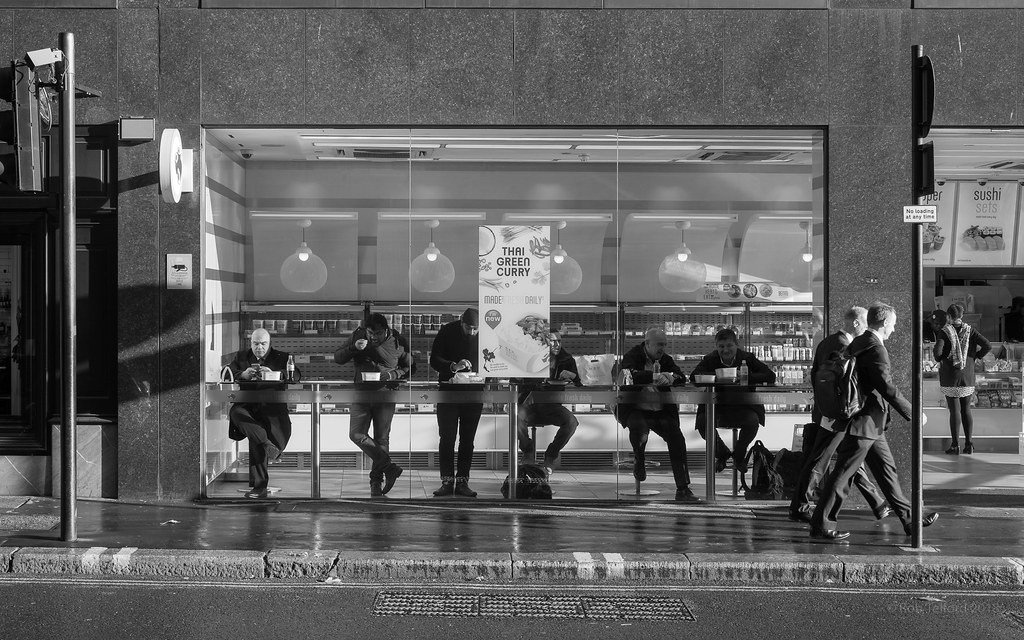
[547,379,571,385]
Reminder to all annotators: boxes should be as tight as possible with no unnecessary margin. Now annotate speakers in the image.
[11,62,46,192]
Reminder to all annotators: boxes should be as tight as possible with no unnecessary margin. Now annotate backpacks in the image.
[741,441,787,502]
[812,340,886,420]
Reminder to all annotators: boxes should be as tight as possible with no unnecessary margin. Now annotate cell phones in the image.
[251,364,261,371]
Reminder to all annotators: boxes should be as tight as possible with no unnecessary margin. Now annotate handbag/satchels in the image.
[775,448,806,499]
[499,464,553,499]
[802,423,819,457]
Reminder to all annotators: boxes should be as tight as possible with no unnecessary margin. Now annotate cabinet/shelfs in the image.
[237,299,815,416]
[922,341,1024,453]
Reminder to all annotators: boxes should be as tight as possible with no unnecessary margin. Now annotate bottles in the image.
[653,359,660,384]
[745,343,794,361]
[740,359,749,386]
[772,364,813,385]
[286,354,295,382]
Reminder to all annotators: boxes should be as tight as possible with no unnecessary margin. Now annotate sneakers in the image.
[522,441,536,466]
[543,453,553,475]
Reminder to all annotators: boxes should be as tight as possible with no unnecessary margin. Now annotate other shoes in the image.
[731,452,749,473]
[713,448,731,471]
[432,480,454,496]
[263,441,281,461]
[382,464,402,493]
[456,482,478,497]
[633,456,647,480]
[945,444,959,455]
[369,478,383,496]
[963,444,975,454]
[244,487,268,498]
[675,488,703,500]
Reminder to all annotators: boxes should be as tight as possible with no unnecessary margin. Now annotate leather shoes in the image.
[904,513,939,536]
[809,526,850,542]
[788,510,814,523]
[875,501,895,521]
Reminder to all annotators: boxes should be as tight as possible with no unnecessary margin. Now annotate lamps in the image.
[658,221,706,291]
[411,220,454,293]
[118,116,156,141]
[281,219,327,293]
[786,222,812,292]
[550,220,582,294]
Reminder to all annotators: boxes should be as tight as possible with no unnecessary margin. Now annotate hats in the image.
[463,309,479,326]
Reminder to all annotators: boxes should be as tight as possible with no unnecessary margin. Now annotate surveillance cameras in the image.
[977,178,988,186]
[936,178,946,187]
[241,149,256,159]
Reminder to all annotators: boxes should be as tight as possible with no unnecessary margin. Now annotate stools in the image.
[715,427,747,496]
[527,422,557,493]
[614,458,661,497]
[236,457,282,492]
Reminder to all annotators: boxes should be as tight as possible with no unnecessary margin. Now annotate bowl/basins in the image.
[261,370,281,381]
[361,371,381,382]
[714,367,737,379]
[694,374,715,383]
[457,372,475,378]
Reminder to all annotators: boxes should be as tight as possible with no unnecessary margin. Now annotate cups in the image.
[768,319,813,336]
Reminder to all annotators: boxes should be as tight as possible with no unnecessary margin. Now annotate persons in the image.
[928,305,992,455]
[809,303,940,541]
[220,329,301,498]
[507,328,584,473]
[689,329,776,473]
[334,312,417,496]
[789,307,895,522]
[614,328,699,501]
[429,308,490,498]
[1005,296,1024,342]
